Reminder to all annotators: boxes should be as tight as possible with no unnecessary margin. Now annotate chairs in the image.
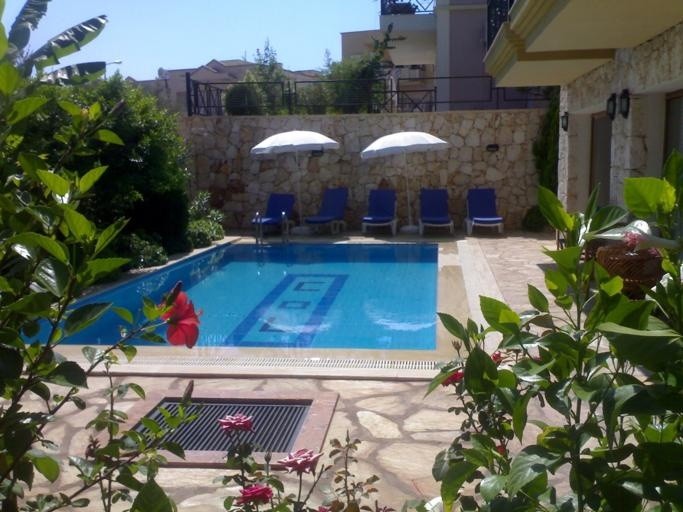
[555,220,664,303]
[248,182,505,238]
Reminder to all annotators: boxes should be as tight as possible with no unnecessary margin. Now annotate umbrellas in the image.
[249,128,340,225]
[360,130,451,224]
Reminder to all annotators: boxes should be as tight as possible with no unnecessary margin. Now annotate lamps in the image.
[560,111,569,132]
[485,143,499,152]
[310,149,324,159]
[605,87,629,121]
